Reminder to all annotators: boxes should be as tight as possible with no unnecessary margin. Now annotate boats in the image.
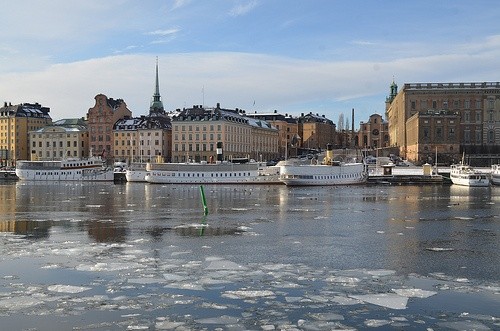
[450,151,490,186]
[491,164,500,184]
[125,160,146,182]
[14,155,114,181]
[277,143,368,187]
[145,152,280,185]
